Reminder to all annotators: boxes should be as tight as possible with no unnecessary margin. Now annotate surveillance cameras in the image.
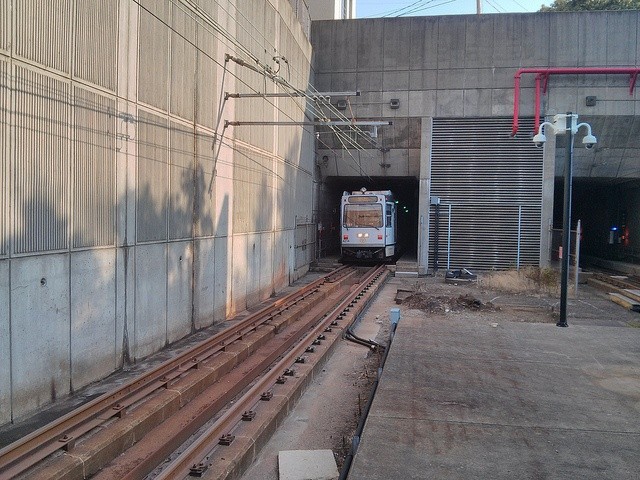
[582,135,598,150]
[533,134,547,149]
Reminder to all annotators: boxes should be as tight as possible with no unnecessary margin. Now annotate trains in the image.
[339,187,400,263]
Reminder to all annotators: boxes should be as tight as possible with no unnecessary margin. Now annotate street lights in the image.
[532,114,598,327]
